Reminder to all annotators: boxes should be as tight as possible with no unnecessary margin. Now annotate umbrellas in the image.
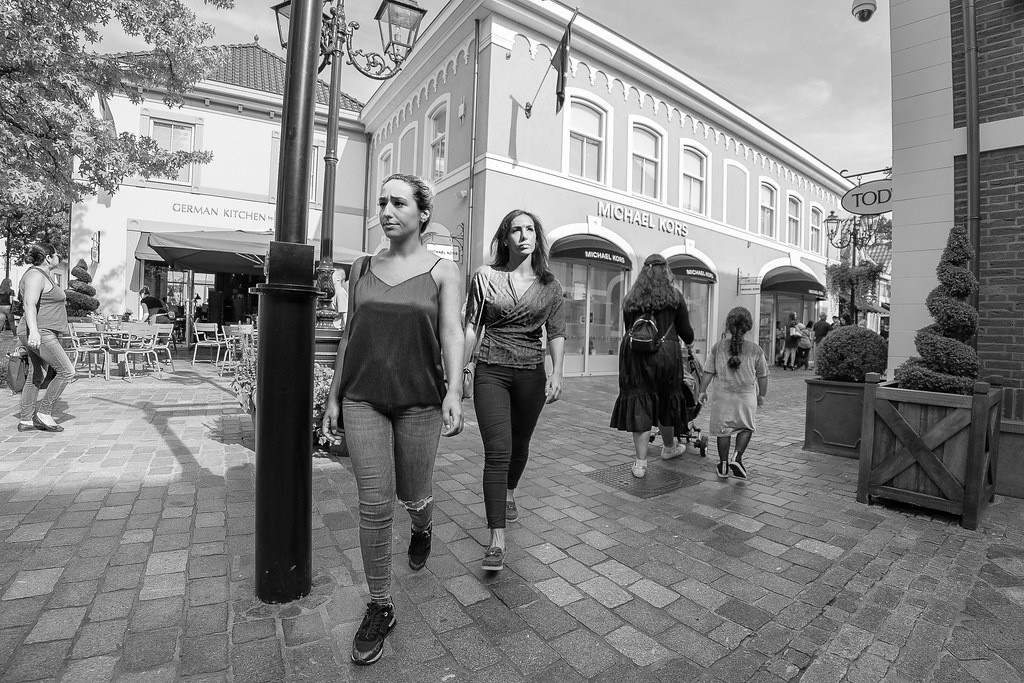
[148,228,373,282]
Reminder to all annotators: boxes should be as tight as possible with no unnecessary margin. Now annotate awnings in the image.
[840,293,890,317]
[760,266,828,300]
[666,255,717,285]
[134,232,164,262]
[551,236,632,271]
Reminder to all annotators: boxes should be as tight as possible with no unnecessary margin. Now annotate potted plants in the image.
[227,320,334,447]
[64,258,100,321]
[803,325,888,460]
[855,224,1002,530]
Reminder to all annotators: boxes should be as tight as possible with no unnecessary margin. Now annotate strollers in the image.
[646,343,708,457]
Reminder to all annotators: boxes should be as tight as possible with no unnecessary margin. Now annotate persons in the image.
[322,174,466,664]
[17,243,75,432]
[139,287,171,364]
[880,322,890,344]
[463,210,568,570]
[0,278,19,340]
[609,253,694,478]
[776,312,852,371]
[698,307,769,478]
[859,319,867,327]
[332,268,348,328]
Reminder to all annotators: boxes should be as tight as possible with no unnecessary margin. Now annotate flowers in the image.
[87,310,105,324]
[124,308,133,314]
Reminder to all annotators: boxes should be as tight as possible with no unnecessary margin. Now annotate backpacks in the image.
[626,305,675,354]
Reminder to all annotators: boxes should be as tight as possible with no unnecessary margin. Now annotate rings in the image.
[31,342,34,343]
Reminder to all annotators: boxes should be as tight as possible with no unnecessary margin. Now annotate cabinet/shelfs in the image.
[564,300,614,327]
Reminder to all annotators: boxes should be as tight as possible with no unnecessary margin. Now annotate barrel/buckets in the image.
[96,324,106,331]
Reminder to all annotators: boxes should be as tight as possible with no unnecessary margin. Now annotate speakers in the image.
[455,189,468,200]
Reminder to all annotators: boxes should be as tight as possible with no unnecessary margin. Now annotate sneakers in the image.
[408,518,432,571]
[350,601,396,665]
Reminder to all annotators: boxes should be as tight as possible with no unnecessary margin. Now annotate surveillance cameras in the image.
[851,0,878,22]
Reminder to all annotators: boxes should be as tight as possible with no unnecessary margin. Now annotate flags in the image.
[551,22,573,114]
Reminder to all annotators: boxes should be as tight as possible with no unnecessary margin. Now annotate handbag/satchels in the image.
[7,352,57,394]
[463,362,475,398]
[790,326,802,338]
[10,300,23,316]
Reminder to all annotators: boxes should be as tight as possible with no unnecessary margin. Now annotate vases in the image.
[124,314,129,321]
[96,324,105,331]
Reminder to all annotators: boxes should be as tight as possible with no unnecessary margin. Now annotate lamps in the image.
[454,190,468,200]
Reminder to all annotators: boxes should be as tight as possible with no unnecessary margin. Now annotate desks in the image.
[80,331,128,376]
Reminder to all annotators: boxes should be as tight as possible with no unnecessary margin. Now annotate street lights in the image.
[270,0,428,447]
[824,210,881,325]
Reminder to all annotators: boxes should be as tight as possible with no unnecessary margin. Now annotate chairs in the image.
[192,317,258,377]
[59,316,175,384]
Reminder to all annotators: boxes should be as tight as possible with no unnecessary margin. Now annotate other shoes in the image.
[790,365,795,371]
[162,359,171,364]
[729,451,747,477]
[481,545,506,570]
[505,496,518,523]
[32,414,64,432]
[17,423,46,432]
[716,460,729,478]
[632,462,647,477]
[783,364,787,370]
[146,361,158,367]
[660,444,686,459]
